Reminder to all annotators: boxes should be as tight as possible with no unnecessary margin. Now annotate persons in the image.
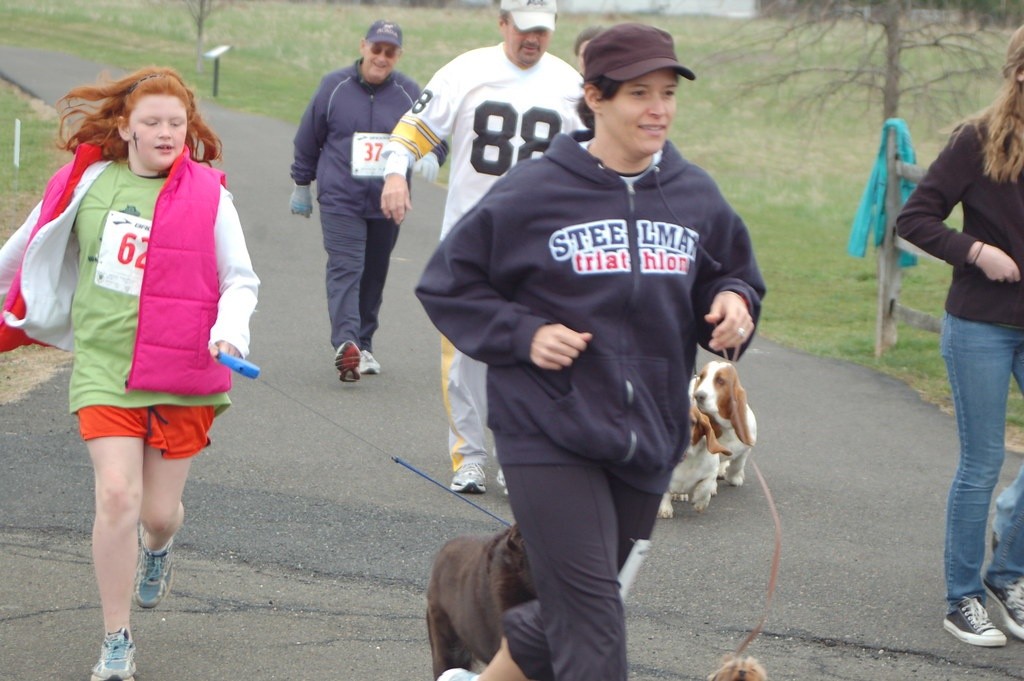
[0,68,261,681]
[379,0,590,493]
[896,22,1024,648]
[289,16,450,383]
[414,22,766,681]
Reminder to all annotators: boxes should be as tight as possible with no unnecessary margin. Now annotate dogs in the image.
[657,408,732,519]
[426,522,537,681]
[687,361,757,487]
[707,656,767,681]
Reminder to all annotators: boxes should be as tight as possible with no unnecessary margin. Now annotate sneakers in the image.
[89,626,137,681]
[944,596,1006,646]
[358,349,382,374]
[135,522,175,609]
[451,462,488,493]
[333,340,362,382]
[497,469,508,490]
[983,575,1024,641]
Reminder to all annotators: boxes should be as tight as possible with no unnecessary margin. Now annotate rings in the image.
[738,328,745,336]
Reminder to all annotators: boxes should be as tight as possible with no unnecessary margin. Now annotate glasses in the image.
[365,40,399,59]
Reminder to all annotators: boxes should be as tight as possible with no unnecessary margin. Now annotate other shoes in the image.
[436,668,479,681]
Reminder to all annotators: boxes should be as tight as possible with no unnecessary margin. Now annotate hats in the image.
[499,0,558,32]
[365,20,403,50]
[584,22,696,82]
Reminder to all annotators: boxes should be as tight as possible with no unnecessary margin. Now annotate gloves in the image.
[415,151,439,180]
[291,183,313,219]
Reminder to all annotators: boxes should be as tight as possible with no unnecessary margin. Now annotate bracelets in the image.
[974,242,985,262]
[736,291,748,307]
